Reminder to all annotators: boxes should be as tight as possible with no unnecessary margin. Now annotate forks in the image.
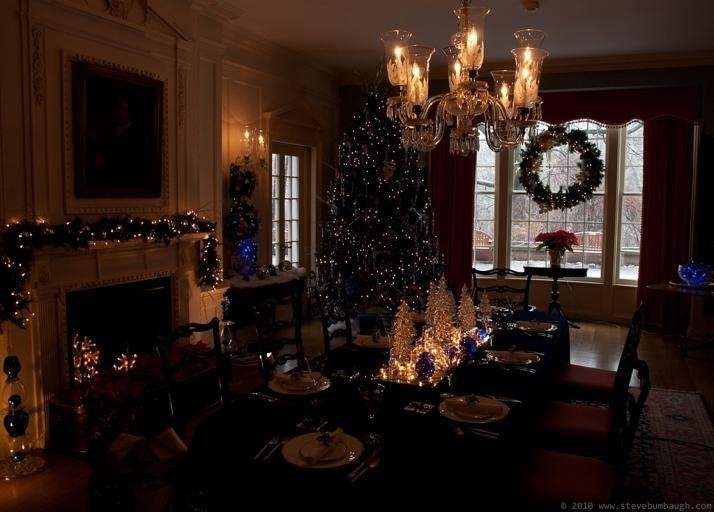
[253,435,279,459]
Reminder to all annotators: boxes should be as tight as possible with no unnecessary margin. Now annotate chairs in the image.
[103,265,650,512]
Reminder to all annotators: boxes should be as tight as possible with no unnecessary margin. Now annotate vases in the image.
[547,251,565,267]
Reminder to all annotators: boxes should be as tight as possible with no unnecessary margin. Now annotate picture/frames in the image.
[61,46,170,215]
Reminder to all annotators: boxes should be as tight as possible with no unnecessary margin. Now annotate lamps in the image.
[238,122,267,170]
[379,1,550,156]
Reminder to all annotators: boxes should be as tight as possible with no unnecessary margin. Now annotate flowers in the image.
[518,127,605,214]
[531,230,578,255]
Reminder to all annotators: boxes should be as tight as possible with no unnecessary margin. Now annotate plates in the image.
[512,319,559,333]
[282,431,363,470]
[270,373,331,395]
[486,350,540,366]
[439,395,509,423]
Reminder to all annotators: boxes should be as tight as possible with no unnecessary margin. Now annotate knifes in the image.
[349,446,385,478]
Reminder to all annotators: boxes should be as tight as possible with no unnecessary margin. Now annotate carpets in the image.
[627,386,714,512]
